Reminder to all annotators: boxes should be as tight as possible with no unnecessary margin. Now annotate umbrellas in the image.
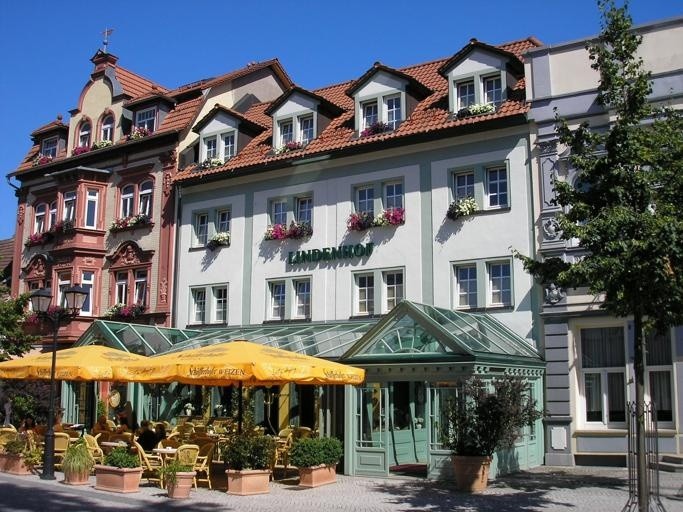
[116,341,368,435]
[0,345,152,426]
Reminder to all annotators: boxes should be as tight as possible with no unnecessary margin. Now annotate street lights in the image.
[29,282,90,480]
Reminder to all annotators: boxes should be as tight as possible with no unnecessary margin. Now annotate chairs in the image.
[0,416,306,492]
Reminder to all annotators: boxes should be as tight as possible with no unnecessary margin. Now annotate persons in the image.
[91,416,167,452]
[18,415,80,439]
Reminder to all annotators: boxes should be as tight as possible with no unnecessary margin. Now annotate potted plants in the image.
[92,427,344,499]
[433,370,552,491]
